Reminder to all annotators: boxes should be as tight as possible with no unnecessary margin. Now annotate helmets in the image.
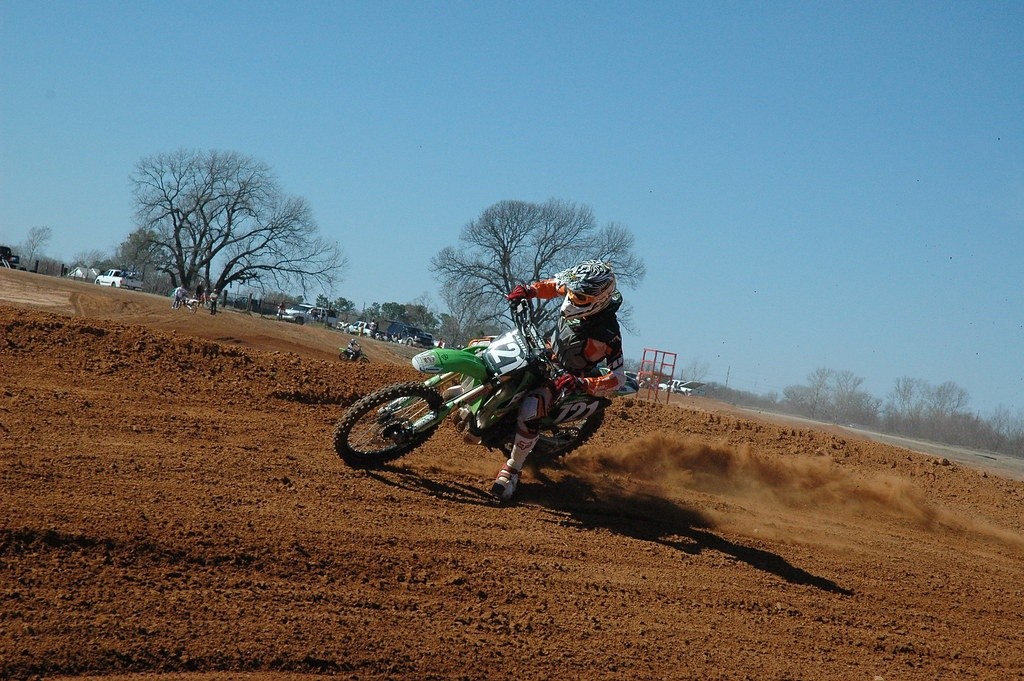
[351,338,356,343]
[560,260,616,320]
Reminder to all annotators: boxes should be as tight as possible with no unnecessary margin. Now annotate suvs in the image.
[281,304,435,350]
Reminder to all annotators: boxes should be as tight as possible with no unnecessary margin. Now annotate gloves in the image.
[555,373,588,397]
[506,283,536,300]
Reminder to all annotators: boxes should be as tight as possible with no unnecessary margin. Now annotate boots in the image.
[492,430,539,499]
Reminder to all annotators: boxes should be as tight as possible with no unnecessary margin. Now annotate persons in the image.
[369,319,376,337]
[312,309,325,321]
[348,339,360,362]
[279,302,285,319]
[171,283,185,309]
[489,259,625,501]
[210,289,218,315]
[437,337,443,349]
[195,281,205,307]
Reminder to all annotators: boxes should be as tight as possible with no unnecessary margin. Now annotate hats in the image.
[214,289,217,291]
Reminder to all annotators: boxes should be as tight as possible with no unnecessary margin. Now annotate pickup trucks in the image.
[623,369,707,397]
[94,269,145,292]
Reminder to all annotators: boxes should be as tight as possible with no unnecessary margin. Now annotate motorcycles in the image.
[175,297,198,314]
[338,344,371,365]
[331,299,640,467]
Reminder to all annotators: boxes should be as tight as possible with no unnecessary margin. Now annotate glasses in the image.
[567,289,596,306]
